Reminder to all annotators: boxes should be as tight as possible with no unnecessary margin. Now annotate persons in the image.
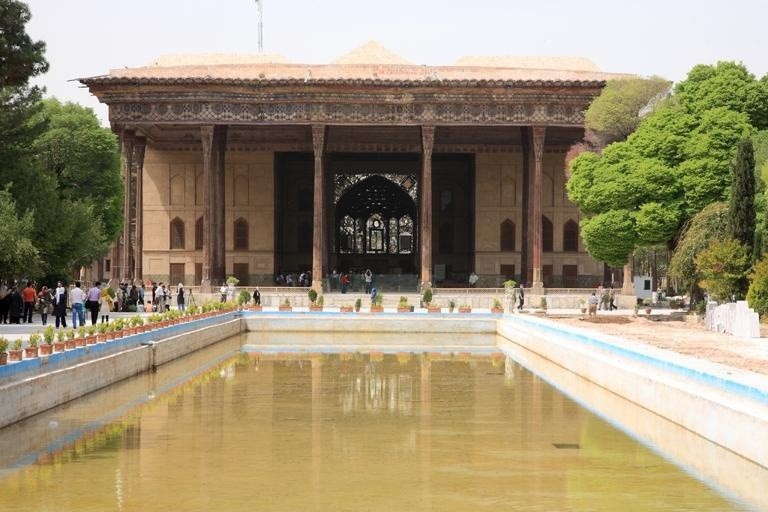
[220,283,226,303]
[370,287,377,307]
[253,287,260,304]
[657,286,663,299]
[597,285,610,311]
[516,283,524,310]
[610,285,618,311]
[587,293,597,315]
[469,272,478,287]
[273,265,373,295]
[1,278,199,328]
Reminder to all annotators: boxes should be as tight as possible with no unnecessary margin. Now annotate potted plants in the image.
[1,299,240,365]
[248,293,508,320]
[539,296,547,311]
[670,300,678,309]
[602,291,610,310]
[642,297,651,314]
[578,299,587,313]
